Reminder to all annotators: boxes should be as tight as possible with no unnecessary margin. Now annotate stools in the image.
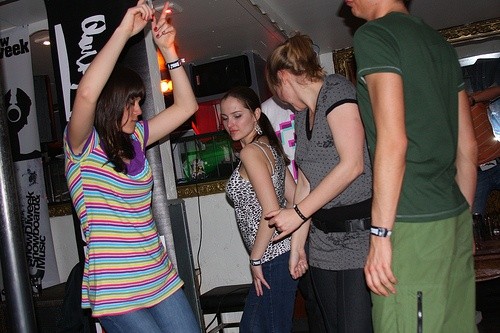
[199,283,254,333]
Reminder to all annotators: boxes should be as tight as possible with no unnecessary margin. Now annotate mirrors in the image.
[332,17,500,144]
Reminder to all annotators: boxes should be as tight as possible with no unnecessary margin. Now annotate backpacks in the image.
[58,255,97,333]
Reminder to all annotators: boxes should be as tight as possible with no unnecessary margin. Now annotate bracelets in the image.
[165,59,182,70]
[370,225,392,237]
[249,259,261,266]
[293,204,307,221]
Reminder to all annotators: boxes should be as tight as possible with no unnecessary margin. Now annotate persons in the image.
[220,87,298,333]
[344,1,478,333]
[263,30,374,333]
[63,0,200,332]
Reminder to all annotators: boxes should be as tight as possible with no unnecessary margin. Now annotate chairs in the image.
[30,199,97,333]
